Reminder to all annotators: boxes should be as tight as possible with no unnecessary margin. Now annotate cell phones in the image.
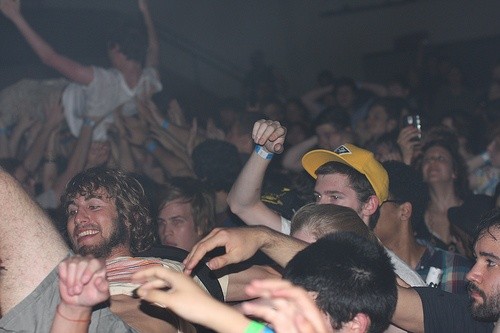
[402,114,422,141]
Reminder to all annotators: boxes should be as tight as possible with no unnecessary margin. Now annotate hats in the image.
[301,143,389,208]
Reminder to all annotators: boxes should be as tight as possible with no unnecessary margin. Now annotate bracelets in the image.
[481,150,493,164]
[144,136,162,152]
[160,118,170,128]
[254,144,275,159]
[83,117,97,127]
[246,319,274,333]
[56,304,93,324]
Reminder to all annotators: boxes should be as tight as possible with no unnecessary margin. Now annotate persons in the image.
[1,38,499,333]
[0,0,163,143]
